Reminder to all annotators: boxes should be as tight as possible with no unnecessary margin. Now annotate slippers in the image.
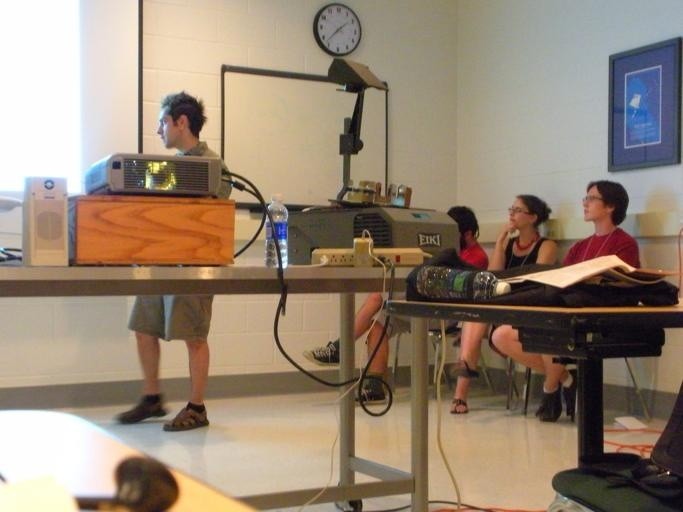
[450,398,468,414]
[452,362,481,376]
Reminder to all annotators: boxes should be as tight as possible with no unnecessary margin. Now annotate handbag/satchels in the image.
[407,248,678,307]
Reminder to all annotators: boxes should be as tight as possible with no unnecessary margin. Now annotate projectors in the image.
[84,153,222,196]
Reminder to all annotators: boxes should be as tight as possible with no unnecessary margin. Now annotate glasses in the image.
[507,207,533,216]
[582,195,605,202]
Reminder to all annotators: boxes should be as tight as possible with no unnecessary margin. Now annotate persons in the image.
[303,206,490,406]
[492,181,641,422]
[450,194,558,414]
[116,90,232,431]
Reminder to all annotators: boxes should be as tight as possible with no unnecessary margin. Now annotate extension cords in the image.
[312,248,424,265]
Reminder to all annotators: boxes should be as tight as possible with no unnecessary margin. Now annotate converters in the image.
[353,238,374,267]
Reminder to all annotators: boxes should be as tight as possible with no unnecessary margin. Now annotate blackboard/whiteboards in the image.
[221,65,389,215]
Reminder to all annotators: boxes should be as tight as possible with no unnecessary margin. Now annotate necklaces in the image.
[516,231,540,250]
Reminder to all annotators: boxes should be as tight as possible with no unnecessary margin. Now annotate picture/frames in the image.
[607,35,680,174]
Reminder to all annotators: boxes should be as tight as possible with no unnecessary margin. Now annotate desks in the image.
[382,298,682,470]
[0,266,430,512]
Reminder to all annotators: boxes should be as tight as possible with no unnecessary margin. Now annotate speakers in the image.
[22,177,69,267]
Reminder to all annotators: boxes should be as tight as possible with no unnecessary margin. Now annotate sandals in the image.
[164,405,208,433]
[116,396,165,423]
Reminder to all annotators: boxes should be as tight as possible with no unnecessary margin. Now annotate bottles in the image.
[416,266,510,301]
[265,194,287,267]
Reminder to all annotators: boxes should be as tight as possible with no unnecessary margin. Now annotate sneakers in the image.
[353,384,385,404]
[539,383,562,421]
[564,369,578,422]
[303,342,340,366]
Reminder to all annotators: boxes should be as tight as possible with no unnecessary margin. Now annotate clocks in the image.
[312,3,362,58]
